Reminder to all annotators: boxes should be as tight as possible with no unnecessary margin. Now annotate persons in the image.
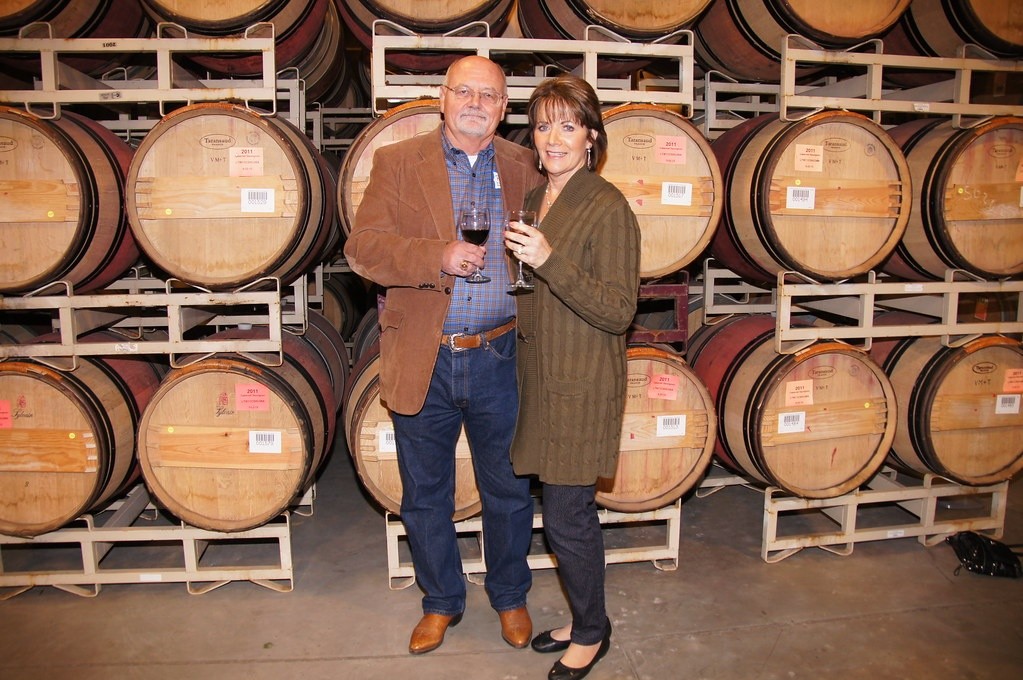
[343,56,548,654]
[502,77,642,680]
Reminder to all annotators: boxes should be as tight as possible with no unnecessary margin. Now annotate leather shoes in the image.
[498,604,533,649]
[409,611,462,654]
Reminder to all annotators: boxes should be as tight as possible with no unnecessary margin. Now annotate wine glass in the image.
[460,209,491,283]
[504,210,536,288]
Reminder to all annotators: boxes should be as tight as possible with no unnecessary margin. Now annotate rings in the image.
[460,261,468,270]
[518,246,524,255]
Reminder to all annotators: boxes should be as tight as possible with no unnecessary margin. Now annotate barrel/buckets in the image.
[0,0,1023,536]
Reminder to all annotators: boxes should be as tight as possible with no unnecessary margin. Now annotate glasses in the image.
[444,85,505,104]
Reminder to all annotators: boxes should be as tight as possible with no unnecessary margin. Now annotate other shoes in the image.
[547,638,610,680]
[531,616,612,653]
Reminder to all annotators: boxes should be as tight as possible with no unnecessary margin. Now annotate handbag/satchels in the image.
[945,531,1022,577]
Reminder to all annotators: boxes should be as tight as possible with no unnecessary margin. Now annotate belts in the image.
[440,317,516,352]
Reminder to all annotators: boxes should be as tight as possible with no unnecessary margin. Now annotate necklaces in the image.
[545,183,552,208]
